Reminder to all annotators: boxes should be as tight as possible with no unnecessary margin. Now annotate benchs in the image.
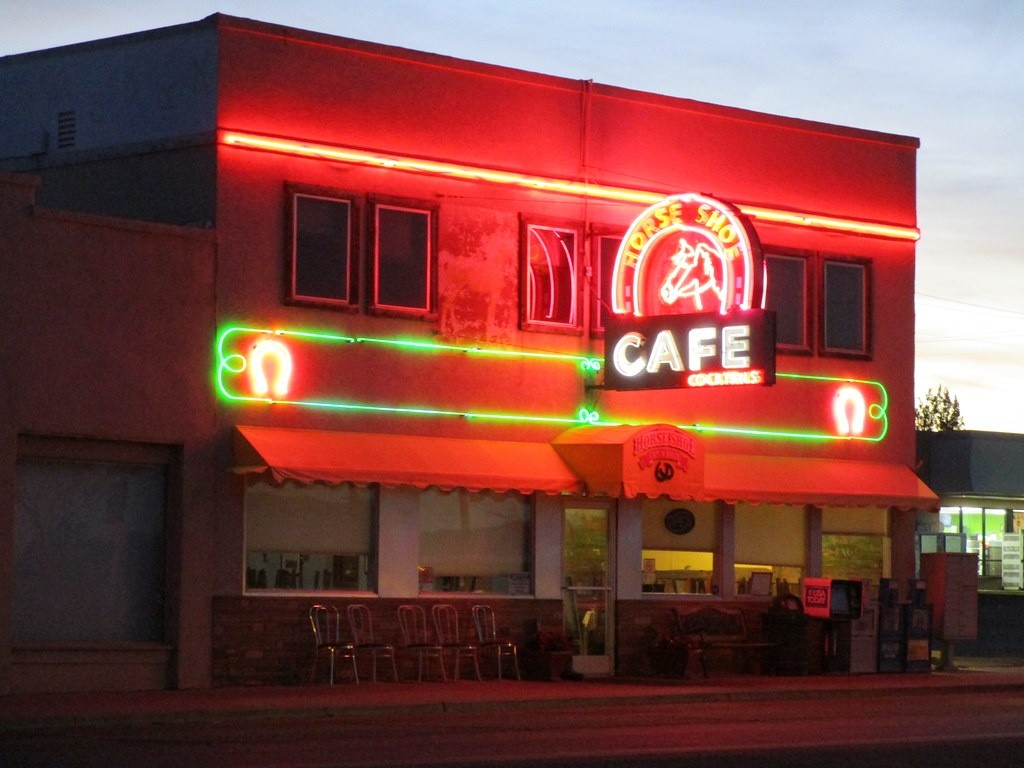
[672,599,785,680]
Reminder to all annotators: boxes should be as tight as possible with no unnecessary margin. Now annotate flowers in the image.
[532,626,571,657]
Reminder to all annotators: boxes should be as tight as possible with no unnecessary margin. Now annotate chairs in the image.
[347,604,400,685]
[309,603,361,685]
[397,605,448,683]
[432,605,483,682]
[471,605,521,682]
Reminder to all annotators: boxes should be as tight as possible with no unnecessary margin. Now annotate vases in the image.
[537,651,571,682]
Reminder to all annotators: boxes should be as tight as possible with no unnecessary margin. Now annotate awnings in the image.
[227,420,942,515]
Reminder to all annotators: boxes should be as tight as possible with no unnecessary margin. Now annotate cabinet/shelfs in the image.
[1001,533,1024,591]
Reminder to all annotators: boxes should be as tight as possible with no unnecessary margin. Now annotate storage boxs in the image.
[802,577,864,620]
